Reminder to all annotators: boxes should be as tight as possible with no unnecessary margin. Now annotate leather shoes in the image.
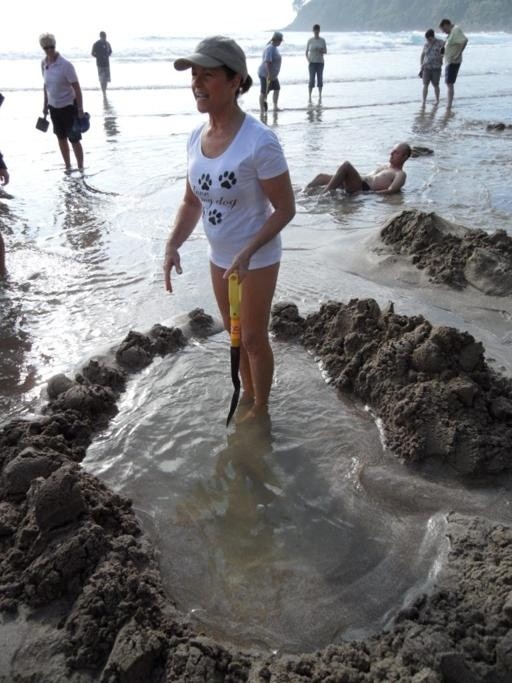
[72,113,89,132]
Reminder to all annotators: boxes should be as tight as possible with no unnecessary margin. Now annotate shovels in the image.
[36,114,49,132]
[263,80,270,112]
[226,272,242,428]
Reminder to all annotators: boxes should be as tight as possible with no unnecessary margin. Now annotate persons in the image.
[163,36,296,432]
[302,143,411,200]
[305,24,327,105]
[0,93,8,279]
[419,28,446,110]
[92,31,112,93]
[258,31,284,113]
[439,18,468,111]
[38,31,84,174]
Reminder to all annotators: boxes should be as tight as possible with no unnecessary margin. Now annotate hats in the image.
[175,36,248,85]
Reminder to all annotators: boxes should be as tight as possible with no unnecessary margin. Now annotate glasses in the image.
[43,45,54,49]
